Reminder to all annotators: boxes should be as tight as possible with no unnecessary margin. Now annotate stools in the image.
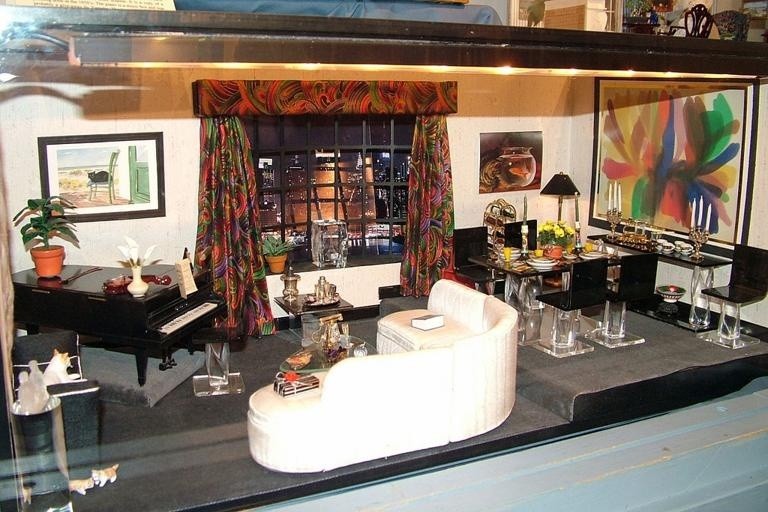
[180,327,246,398]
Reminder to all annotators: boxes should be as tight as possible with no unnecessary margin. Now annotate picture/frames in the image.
[36,131,167,224]
[588,75,760,258]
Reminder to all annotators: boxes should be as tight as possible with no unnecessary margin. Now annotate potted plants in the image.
[11,193,81,278]
[258,235,297,273]
[624,0,652,25]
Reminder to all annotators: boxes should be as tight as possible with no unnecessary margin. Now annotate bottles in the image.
[29,360,46,390]
[319,276,325,286]
[328,284,335,301]
[315,285,319,297]
[342,324,350,347]
[324,282,330,297]
[585,240,592,252]
[18,371,48,412]
[318,287,324,304]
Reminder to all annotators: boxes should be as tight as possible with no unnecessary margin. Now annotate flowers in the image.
[117,235,163,268]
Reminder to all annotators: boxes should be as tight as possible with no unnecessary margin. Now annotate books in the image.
[47,380,100,397]
[411,313,444,331]
[175,257,198,301]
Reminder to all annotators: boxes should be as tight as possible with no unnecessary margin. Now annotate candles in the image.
[705,202,712,232]
[608,178,622,212]
[688,197,696,228]
[697,195,704,226]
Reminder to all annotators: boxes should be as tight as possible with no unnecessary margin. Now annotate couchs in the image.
[245,278,519,474]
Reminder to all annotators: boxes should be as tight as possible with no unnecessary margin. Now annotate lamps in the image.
[539,171,581,220]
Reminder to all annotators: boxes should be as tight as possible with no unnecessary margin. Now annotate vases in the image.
[127,267,149,298]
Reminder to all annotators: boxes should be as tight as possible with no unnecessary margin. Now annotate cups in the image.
[535,250,543,257]
[681,244,694,252]
[504,247,511,260]
[657,239,668,247]
[505,262,510,270]
[663,243,675,251]
[354,347,368,357]
[674,241,685,249]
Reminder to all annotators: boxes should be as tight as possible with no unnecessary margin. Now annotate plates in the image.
[527,262,558,269]
[579,254,598,260]
[564,256,576,259]
[501,258,518,262]
[663,252,674,254]
[303,296,340,306]
[584,252,601,256]
[500,254,522,257]
[532,257,555,263]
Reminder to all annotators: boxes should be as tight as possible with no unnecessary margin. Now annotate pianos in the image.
[8,266,226,392]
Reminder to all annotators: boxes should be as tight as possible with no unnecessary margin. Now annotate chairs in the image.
[702,244,768,350]
[454,219,733,358]
[11,330,101,456]
[657,3,713,38]
